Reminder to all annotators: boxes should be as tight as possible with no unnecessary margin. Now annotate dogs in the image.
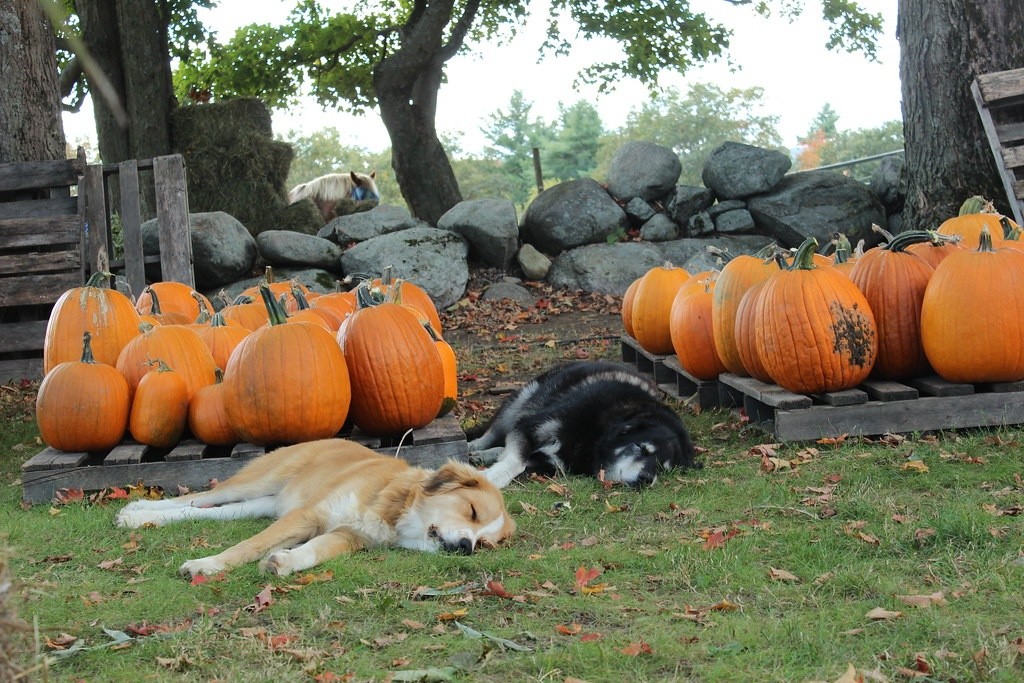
[112,437,519,580]
[461,359,703,490]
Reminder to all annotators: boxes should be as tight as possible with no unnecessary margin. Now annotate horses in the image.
[287,169,380,206]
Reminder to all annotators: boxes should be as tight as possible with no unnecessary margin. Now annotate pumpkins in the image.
[622,195,1024,394]
[35,265,457,446]
